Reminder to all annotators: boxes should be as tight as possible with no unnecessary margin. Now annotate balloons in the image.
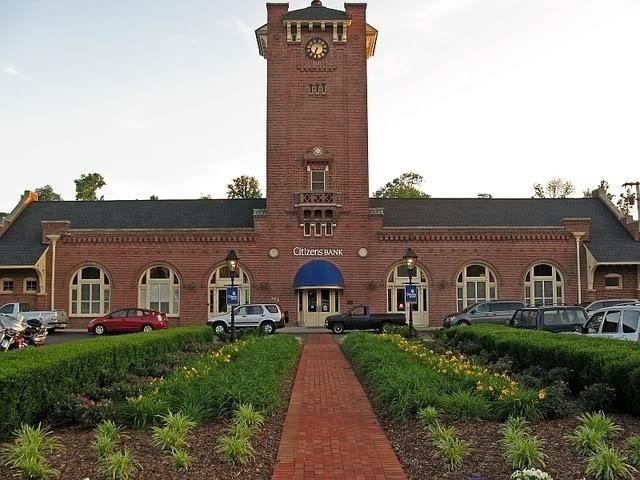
[305,37,329,60]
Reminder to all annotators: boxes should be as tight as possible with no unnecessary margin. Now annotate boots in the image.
[557,306,640,342]
[206,301,289,335]
[504,303,589,333]
[578,299,639,316]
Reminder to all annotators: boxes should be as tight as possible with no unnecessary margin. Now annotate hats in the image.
[88,308,168,334]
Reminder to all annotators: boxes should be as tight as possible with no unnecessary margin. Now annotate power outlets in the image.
[325,305,405,335]
[0,301,68,335]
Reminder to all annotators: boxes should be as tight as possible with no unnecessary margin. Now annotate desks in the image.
[225,250,239,339]
[404,247,417,334]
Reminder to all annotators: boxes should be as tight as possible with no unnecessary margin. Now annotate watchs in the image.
[0,312,48,353]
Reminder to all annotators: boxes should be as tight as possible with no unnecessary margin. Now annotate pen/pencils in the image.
[441,300,526,327]
